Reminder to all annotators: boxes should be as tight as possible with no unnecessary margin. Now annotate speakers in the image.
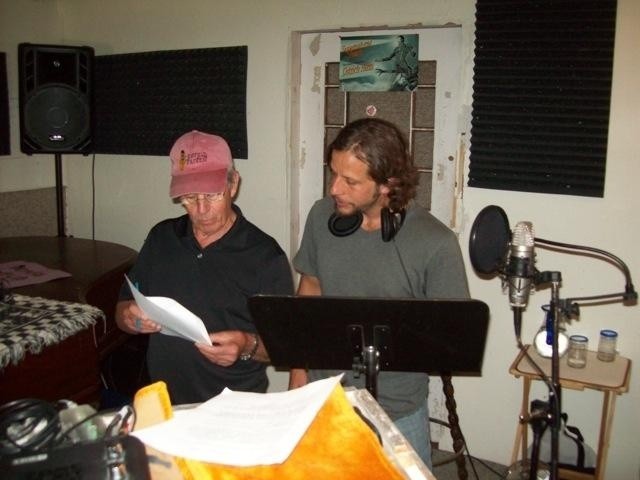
[17,42,94,154]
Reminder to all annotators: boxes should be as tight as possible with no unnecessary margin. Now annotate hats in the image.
[168,129,234,199]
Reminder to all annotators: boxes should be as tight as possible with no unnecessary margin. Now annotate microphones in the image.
[508,221,534,334]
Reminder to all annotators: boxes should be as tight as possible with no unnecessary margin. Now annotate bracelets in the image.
[240,333,260,362]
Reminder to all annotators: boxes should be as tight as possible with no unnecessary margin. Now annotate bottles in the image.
[597,329,619,363]
[567,333,589,369]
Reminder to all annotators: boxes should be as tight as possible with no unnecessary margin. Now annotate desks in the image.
[0,232,139,405]
[510,345,632,480]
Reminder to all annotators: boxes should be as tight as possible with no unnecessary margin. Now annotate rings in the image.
[134,320,142,328]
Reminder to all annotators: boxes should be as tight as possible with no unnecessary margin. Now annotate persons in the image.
[116,128,296,404]
[375,35,416,66]
[288,117,471,473]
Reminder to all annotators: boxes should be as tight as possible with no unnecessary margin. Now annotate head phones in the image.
[327,199,405,242]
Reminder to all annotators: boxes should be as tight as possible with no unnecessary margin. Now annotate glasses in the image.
[174,194,224,205]
